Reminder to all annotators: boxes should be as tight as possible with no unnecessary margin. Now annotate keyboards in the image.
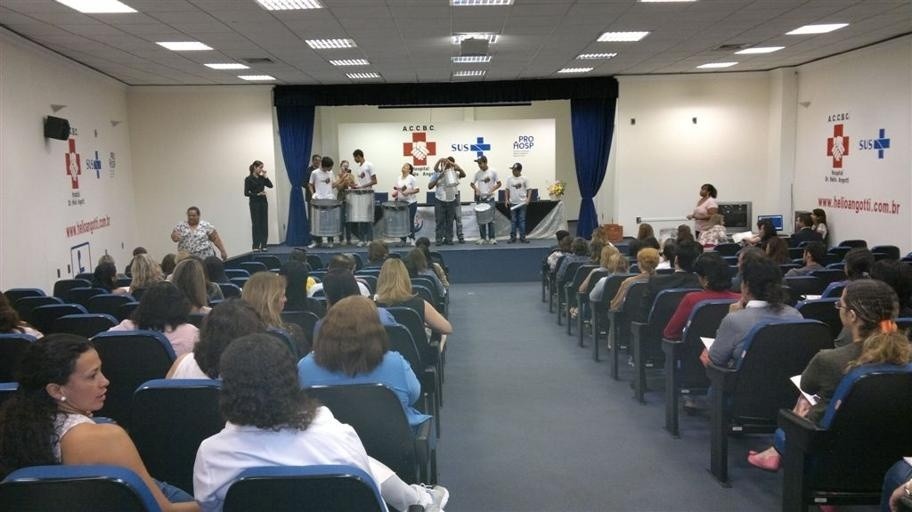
[777,233,789,237]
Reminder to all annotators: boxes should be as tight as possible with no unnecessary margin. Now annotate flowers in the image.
[547,180,566,196]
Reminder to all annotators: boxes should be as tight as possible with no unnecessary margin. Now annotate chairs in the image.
[499,190,510,201]
[528,189,538,200]
[427,192,436,202]
[374,193,388,203]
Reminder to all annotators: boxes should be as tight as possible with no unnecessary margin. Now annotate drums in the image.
[309,200,343,237]
[344,189,375,225]
[380,201,412,238]
[444,169,459,187]
[474,202,494,225]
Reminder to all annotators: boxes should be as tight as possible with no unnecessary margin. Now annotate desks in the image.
[374,200,569,243]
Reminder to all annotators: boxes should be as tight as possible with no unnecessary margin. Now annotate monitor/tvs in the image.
[716,201,752,235]
[757,214,783,231]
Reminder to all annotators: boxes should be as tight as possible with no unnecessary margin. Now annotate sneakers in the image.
[475,239,496,245]
[253,248,267,252]
[747,450,780,471]
[570,308,578,318]
[628,357,654,367]
[414,486,449,512]
[507,239,529,243]
[435,238,464,246]
[307,240,372,249]
[395,238,416,247]
[820,503,835,512]
[682,402,696,416]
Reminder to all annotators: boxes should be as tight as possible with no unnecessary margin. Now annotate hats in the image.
[511,163,522,170]
[474,156,486,162]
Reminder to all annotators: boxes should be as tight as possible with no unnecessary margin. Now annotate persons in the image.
[434,155,467,244]
[470,154,503,246]
[303,150,378,246]
[393,163,420,247]
[504,162,532,244]
[879,458,912,512]
[686,183,719,238]
[193,334,449,512]
[242,160,276,249]
[4,333,200,511]
[747,279,912,470]
[87,236,452,425]
[0,291,44,339]
[171,207,228,260]
[546,208,912,415]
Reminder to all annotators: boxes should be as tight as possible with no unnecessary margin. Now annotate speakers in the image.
[45,115,71,140]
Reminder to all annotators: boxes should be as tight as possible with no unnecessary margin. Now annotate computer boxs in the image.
[795,210,812,234]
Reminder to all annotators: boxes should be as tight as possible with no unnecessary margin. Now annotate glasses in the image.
[834,301,851,312]
